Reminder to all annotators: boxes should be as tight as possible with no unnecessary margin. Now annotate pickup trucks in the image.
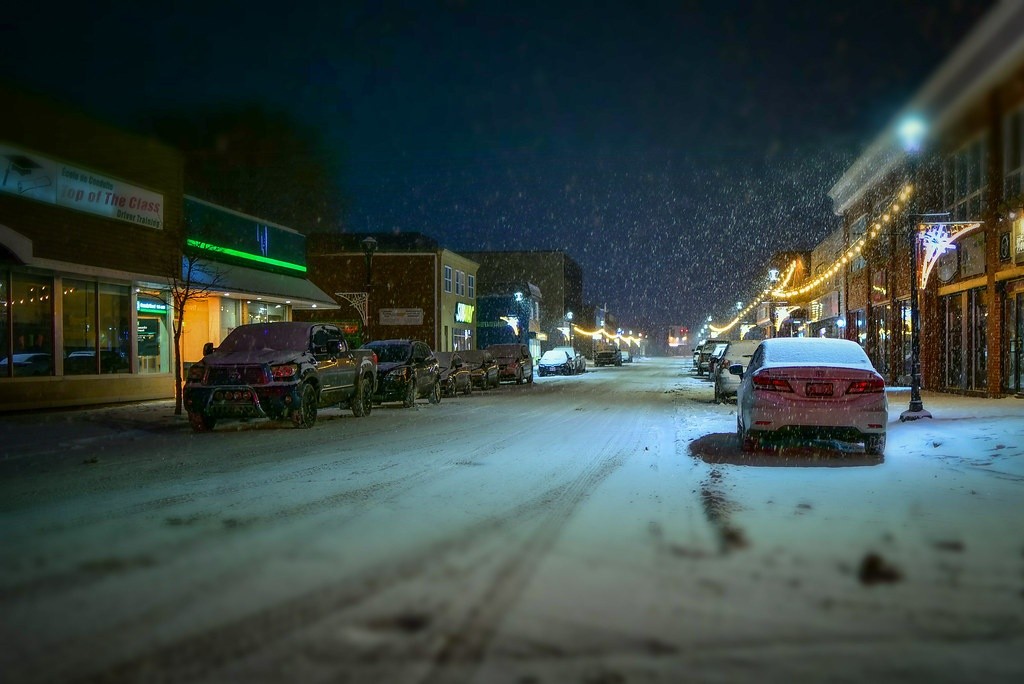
[183,321,379,434]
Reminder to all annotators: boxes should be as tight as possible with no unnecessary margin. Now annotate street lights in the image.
[361,234,381,344]
[900,118,934,423]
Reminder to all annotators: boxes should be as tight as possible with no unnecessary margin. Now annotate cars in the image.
[621,349,633,364]
[692,343,705,365]
[709,336,765,404]
[708,343,730,378]
[693,339,731,376]
[459,349,501,390]
[554,347,587,375]
[537,350,574,377]
[726,337,890,457]
[432,351,472,397]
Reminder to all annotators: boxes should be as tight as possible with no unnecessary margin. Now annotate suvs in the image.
[593,345,623,367]
[485,343,534,385]
[358,338,442,408]
